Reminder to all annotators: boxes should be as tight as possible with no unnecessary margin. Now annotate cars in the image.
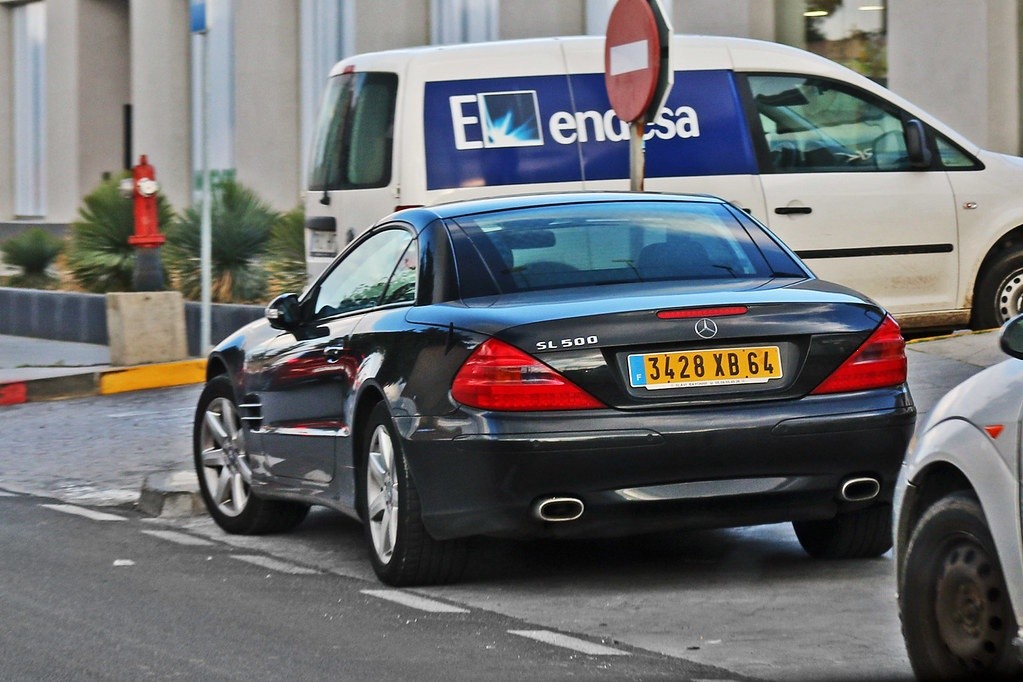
[188,190,920,590]
[890,308,1023,682]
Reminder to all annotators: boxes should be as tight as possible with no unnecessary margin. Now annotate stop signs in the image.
[601,0,661,123]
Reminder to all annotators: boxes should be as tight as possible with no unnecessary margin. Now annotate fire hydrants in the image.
[127,154,166,249]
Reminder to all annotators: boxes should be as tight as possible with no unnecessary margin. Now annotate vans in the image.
[299,30,1023,332]
